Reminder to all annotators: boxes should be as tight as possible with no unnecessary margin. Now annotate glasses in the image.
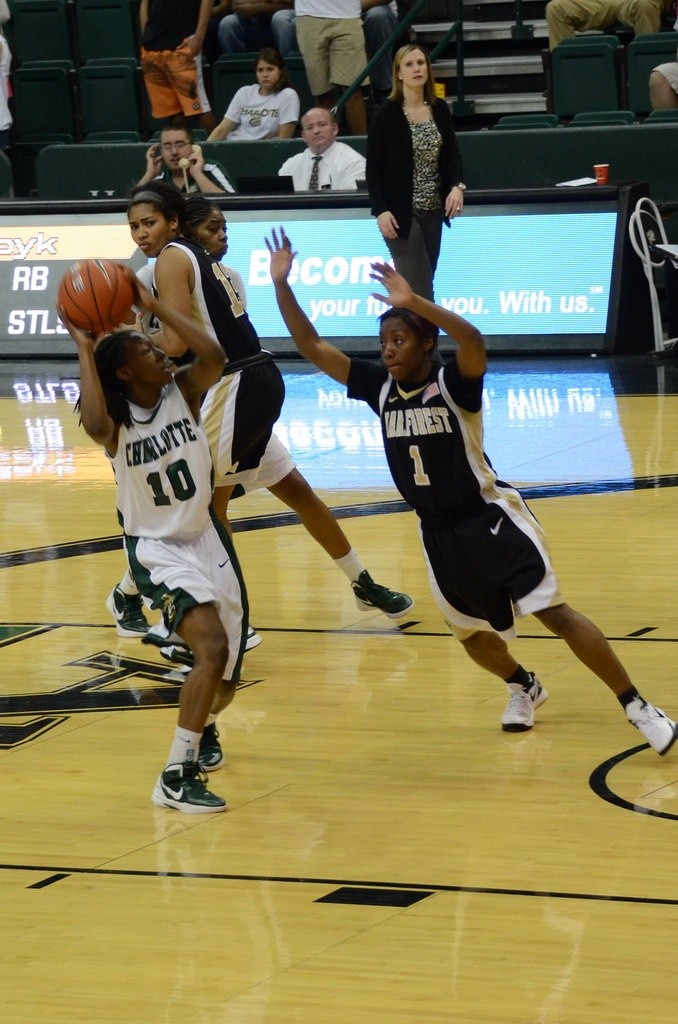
[159,142,191,152]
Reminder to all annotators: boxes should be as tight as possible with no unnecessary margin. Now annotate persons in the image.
[295,0,371,137]
[105,181,413,664]
[139,0,214,144]
[278,108,367,192]
[264,225,678,758]
[0,0,11,158]
[542,0,678,114]
[58,259,248,814]
[133,122,239,193]
[214,0,398,94]
[366,44,465,306]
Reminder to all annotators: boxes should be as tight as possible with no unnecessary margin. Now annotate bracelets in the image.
[455,182,466,191]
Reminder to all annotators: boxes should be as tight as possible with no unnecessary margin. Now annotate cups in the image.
[593,163,609,185]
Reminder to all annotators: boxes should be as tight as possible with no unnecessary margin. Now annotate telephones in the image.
[178,144,202,169]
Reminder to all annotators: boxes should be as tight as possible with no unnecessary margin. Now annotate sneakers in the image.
[500,670,549,732]
[625,697,678,757]
[151,761,227,813]
[351,569,414,619]
[198,721,224,771]
[105,582,153,638]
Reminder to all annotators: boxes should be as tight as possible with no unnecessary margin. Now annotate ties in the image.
[308,156,323,191]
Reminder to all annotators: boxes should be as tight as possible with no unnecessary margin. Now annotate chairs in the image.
[0,0,678,195]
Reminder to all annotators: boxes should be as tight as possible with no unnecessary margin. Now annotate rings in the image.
[456,207,461,211]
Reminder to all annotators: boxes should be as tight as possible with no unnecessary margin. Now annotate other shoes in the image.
[142,623,191,649]
[243,625,263,650]
[160,644,195,668]
